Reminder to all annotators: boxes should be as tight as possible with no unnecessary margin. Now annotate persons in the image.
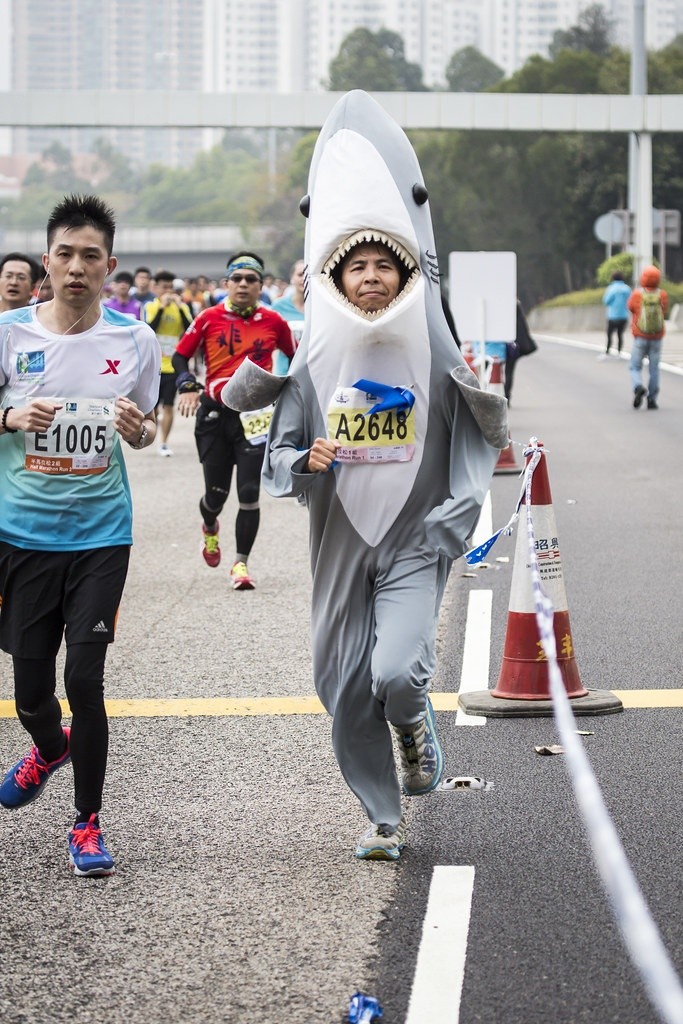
[257,87,507,863]
[596,271,632,359]
[170,251,299,591]
[0,193,166,879]
[626,265,667,411]
[0,253,305,463]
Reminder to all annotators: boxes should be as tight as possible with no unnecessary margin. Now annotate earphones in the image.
[47,265,51,273]
[106,268,108,276]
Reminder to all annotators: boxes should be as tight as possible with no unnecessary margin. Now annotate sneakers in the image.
[68,822,115,876]
[355,815,407,860]
[202,519,221,567]
[0,727,73,810]
[394,695,446,794]
[230,561,256,590]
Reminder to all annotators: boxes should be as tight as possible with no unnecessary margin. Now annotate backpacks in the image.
[635,287,663,334]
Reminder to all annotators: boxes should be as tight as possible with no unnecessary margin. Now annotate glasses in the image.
[227,273,261,283]
[0,272,32,282]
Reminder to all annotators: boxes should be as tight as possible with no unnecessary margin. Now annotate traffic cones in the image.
[463,348,525,474]
[457,441,623,719]
